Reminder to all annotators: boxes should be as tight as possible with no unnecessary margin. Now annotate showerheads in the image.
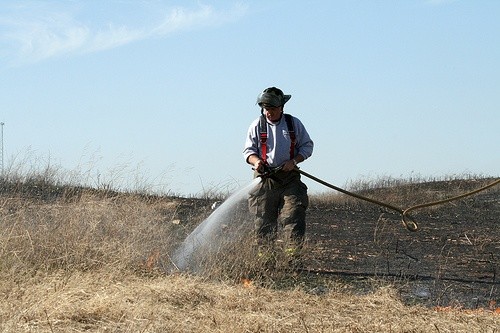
[256,163,284,184]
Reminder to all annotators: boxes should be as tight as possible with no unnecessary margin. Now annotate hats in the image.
[258,87,291,108]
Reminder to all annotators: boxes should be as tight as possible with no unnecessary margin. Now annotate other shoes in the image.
[289,260,302,268]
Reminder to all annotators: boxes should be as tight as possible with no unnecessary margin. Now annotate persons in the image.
[243,87,314,271]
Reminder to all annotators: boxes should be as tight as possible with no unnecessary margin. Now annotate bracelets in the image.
[292,159,298,165]
